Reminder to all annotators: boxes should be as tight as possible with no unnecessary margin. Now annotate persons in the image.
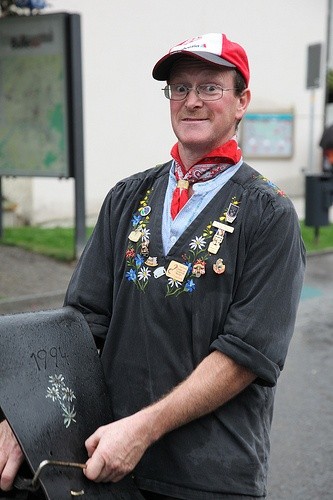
[0,31,308,500]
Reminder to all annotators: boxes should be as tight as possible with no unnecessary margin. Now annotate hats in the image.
[152,32,250,88]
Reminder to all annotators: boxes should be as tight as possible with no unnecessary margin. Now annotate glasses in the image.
[161,82,242,101]
